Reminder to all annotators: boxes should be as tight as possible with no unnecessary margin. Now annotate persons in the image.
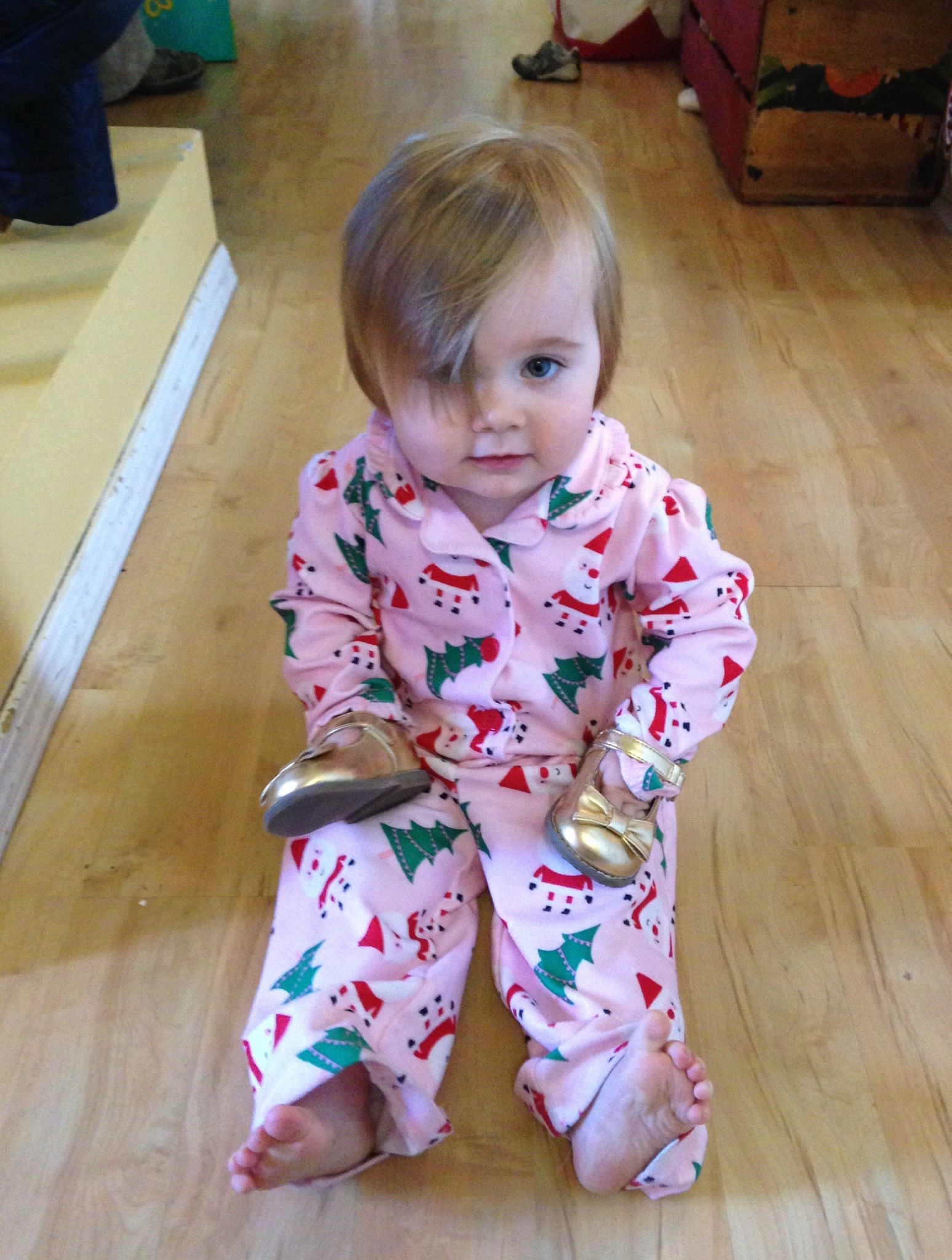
[220,122,758,1205]
[92,9,205,106]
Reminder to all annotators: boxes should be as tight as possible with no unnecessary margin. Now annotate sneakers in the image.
[512,40,582,83]
[133,47,206,93]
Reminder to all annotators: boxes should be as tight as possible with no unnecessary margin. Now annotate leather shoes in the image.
[259,712,432,838]
[544,728,686,889]
